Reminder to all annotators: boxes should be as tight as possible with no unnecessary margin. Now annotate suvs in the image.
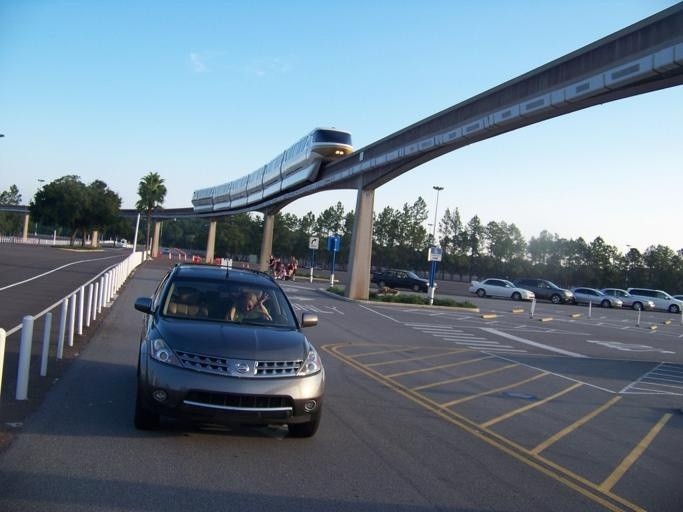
[131,259,328,442]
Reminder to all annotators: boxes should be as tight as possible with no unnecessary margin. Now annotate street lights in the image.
[32,178,46,236]
[426,185,444,241]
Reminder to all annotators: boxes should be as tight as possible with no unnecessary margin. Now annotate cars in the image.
[465,277,682,313]
[369,264,439,294]
[162,247,169,253]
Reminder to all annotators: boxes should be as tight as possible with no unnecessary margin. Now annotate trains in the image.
[190,124,355,214]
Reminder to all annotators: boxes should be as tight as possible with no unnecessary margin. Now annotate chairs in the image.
[167,286,209,317]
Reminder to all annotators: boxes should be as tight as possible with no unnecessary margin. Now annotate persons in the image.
[224,291,272,322]
[267,254,299,282]
